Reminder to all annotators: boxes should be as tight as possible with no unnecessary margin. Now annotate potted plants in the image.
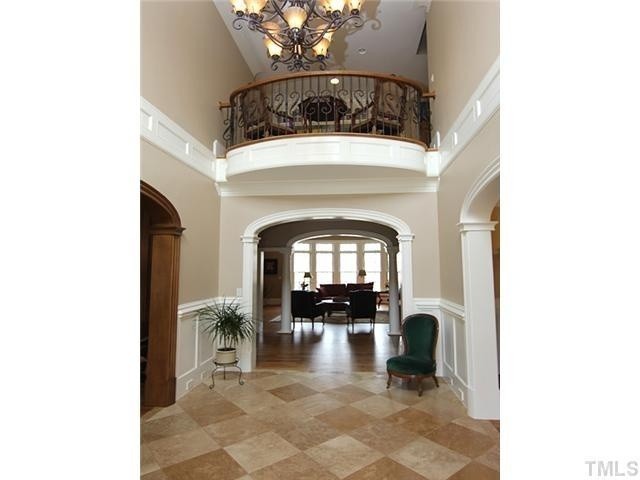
[192,296,262,364]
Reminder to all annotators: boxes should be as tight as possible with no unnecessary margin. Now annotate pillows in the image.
[316,287,330,296]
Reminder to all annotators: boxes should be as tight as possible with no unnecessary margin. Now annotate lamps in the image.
[230,0,365,73]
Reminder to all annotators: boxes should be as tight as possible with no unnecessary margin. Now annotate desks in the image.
[298,95,347,132]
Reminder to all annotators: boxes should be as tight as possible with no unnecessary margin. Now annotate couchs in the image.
[316,282,374,311]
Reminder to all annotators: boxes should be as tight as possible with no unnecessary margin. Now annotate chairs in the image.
[240,85,296,141]
[348,77,407,137]
[291,290,377,328]
[385,313,440,397]
